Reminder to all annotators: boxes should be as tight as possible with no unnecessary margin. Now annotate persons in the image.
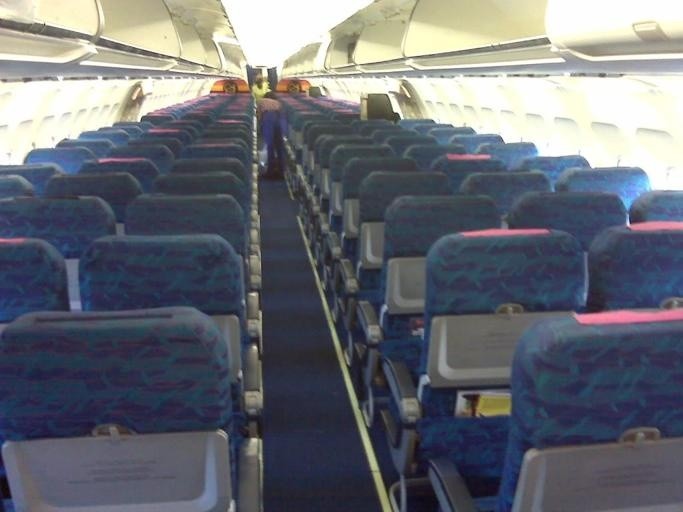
[258,92,288,178]
[252,73,271,106]
